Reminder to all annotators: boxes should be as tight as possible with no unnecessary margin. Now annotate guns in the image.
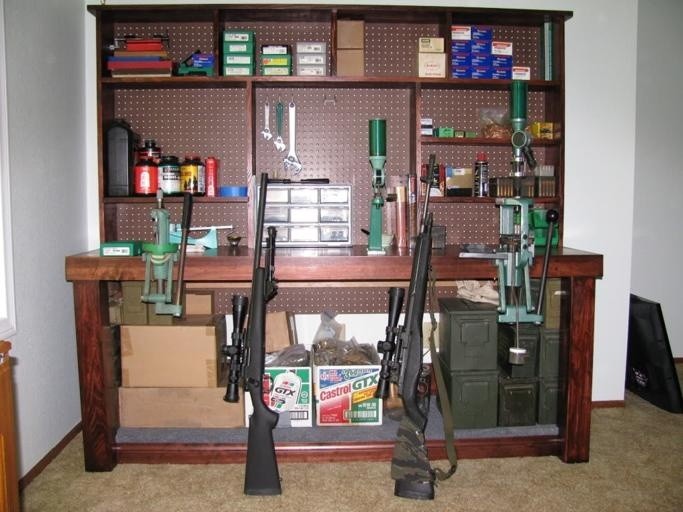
[221,173,282,495]
[374,153,436,500]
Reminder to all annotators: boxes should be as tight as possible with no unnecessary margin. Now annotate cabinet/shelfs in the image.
[84,3,574,249]
[63,248,605,471]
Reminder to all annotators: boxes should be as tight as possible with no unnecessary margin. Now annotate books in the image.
[106,37,174,78]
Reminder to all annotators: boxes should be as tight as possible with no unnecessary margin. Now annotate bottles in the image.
[473,151,489,198]
[133,139,218,197]
